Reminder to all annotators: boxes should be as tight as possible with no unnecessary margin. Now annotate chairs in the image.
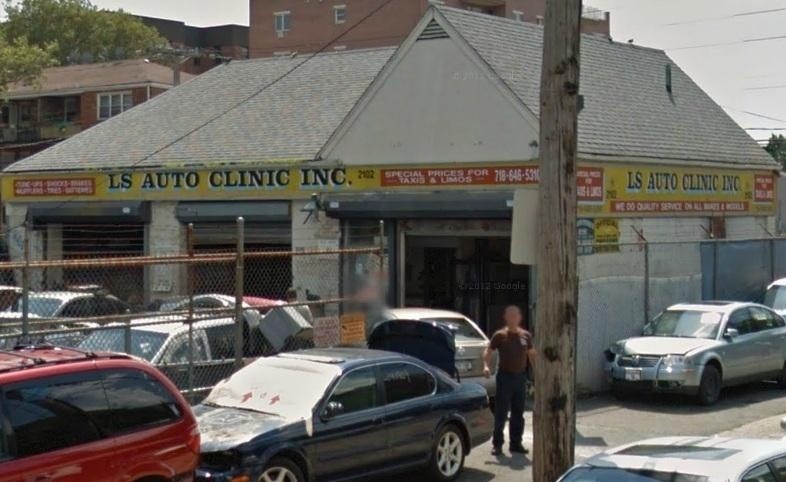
[348,375,425,410]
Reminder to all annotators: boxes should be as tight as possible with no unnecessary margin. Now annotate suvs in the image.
[0,342,202,481]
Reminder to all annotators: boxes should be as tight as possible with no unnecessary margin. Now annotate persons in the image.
[285,287,314,324]
[480,307,535,456]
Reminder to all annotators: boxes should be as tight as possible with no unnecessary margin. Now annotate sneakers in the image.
[491,444,503,454]
[510,442,530,454]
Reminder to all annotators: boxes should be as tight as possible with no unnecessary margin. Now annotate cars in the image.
[383,306,501,413]
[0,292,135,318]
[604,297,785,408]
[190,344,497,481]
[556,434,786,482]
[74,311,266,407]
